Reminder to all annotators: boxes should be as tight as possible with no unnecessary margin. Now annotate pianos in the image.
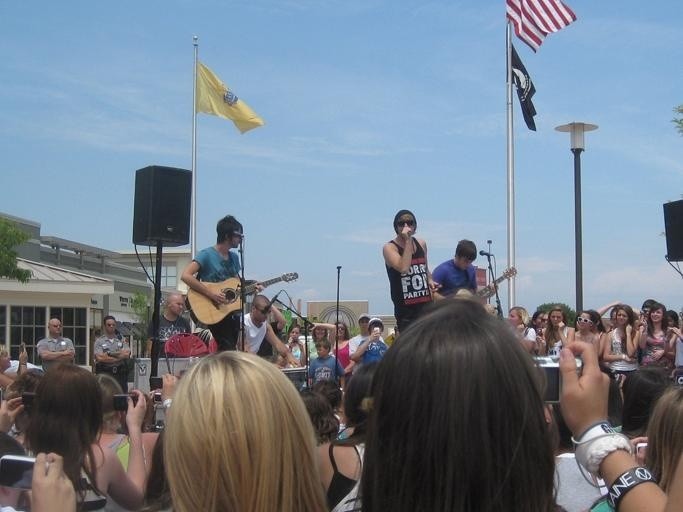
[280,367,307,382]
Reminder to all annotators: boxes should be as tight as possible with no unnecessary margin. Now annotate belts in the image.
[107,366,124,373]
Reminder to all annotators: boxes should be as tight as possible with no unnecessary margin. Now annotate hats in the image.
[357,313,384,332]
[393,209,417,232]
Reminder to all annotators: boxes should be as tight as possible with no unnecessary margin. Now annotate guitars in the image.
[185,272,298,330]
[454,267,517,313]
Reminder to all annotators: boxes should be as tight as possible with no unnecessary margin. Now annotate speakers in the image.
[663,199,683,261]
[132,165,192,246]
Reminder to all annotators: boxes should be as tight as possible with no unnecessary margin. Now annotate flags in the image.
[505,0,577,53]
[512,43,538,132]
[195,61,264,133]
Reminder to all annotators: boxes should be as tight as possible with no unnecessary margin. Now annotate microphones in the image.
[480,251,492,258]
[265,291,281,310]
[233,230,245,239]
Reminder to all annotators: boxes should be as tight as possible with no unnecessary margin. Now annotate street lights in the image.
[550,120,603,322]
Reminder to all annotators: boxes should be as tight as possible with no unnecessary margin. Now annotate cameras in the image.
[150,376,165,390]
[153,394,161,402]
[373,327,379,332]
[533,355,581,405]
[23,394,35,408]
[2,456,52,489]
[113,394,133,412]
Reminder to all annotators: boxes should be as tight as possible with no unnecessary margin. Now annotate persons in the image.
[383,210,444,333]
[180,215,263,352]
[0,289,683,512]
[432,239,496,301]
